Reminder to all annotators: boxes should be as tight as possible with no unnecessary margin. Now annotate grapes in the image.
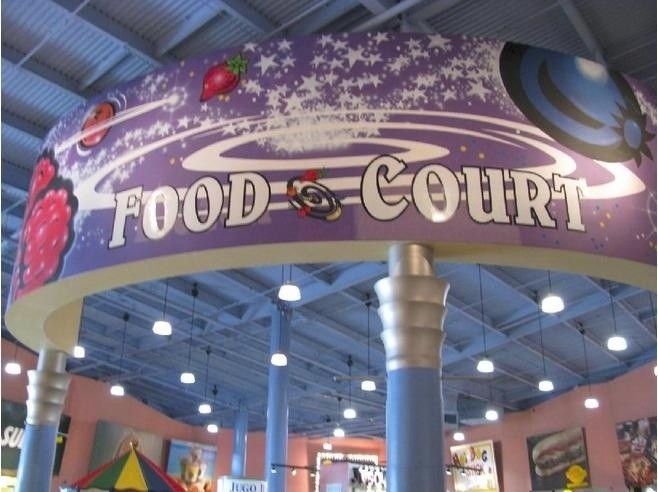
[12,153,74,298]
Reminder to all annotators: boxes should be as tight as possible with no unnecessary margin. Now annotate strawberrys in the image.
[300,167,323,182]
[201,57,248,104]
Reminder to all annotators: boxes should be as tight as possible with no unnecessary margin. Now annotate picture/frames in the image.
[448,440,500,492]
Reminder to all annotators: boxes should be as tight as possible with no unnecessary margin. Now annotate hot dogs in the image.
[531,428,587,477]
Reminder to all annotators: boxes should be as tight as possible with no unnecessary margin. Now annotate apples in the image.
[80,103,113,147]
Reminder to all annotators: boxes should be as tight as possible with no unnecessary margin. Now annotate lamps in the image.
[453,431,465,442]
[5,339,23,378]
[197,345,212,415]
[278,262,302,303]
[72,320,87,360]
[207,385,219,433]
[270,265,288,367]
[151,279,174,337]
[577,322,601,410]
[606,279,628,352]
[541,270,566,315]
[485,372,498,421]
[179,282,199,384]
[474,264,494,375]
[322,417,333,450]
[531,289,555,392]
[106,312,131,396]
[332,395,345,439]
[361,290,377,392]
[342,352,357,419]
